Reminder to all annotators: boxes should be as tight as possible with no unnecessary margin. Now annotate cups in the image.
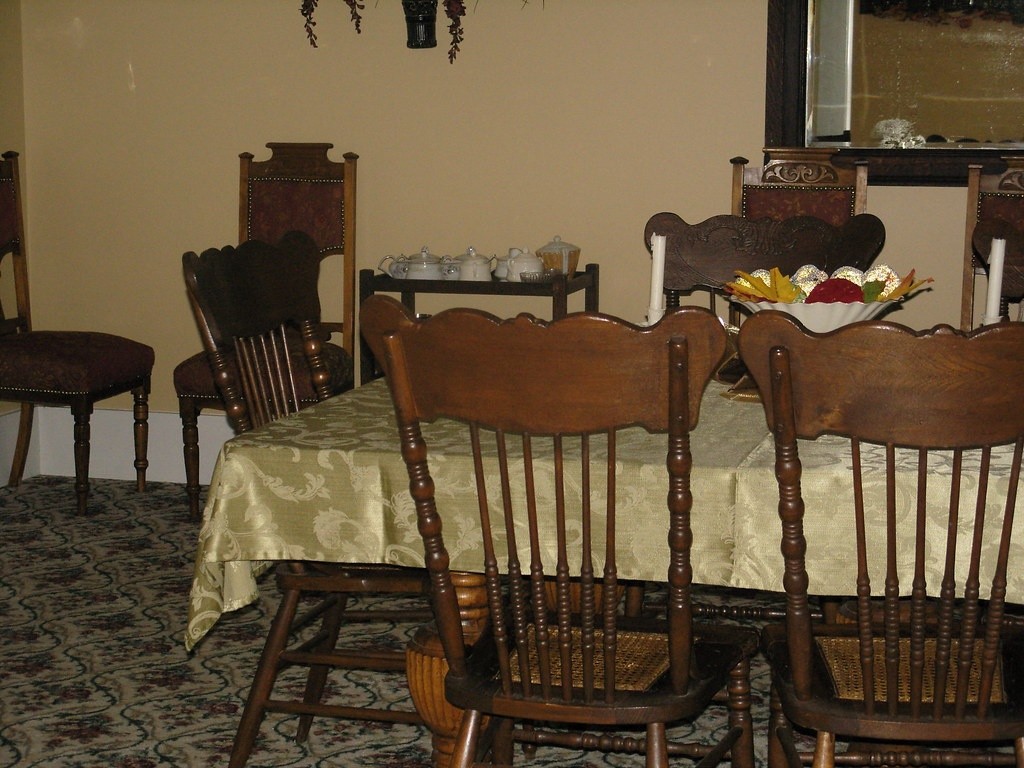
[507,247,543,282]
[453,246,496,281]
[378,242,462,281]
[494,248,511,282]
[535,236,581,281]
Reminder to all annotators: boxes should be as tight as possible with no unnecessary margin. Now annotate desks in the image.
[184,377,1024,768]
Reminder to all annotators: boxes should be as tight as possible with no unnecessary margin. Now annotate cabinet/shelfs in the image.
[359,261,598,383]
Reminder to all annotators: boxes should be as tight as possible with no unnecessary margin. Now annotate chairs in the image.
[0,142,1024,768]
